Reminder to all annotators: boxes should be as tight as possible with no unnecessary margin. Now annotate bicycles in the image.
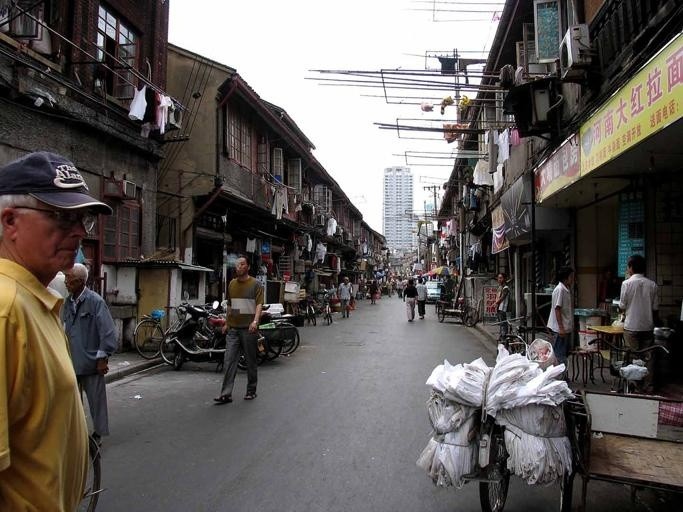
[302,293,317,326]
[491,313,526,356]
[128,299,185,360]
[460,335,571,511]
[320,288,334,326]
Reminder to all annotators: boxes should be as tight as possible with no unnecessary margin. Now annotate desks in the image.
[585,322,639,395]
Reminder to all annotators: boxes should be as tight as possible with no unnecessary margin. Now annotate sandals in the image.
[214,394,232,403]
[244,393,257,400]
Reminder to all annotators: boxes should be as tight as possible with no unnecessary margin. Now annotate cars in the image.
[424,281,443,301]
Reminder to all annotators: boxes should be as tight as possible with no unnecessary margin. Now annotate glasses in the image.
[12,206,97,232]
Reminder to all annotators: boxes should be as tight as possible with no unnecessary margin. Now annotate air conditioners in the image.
[556,23,590,81]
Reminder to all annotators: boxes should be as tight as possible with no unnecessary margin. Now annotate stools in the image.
[570,344,609,387]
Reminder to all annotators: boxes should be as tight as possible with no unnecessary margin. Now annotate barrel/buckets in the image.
[586,316,601,330]
[578,331,588,350]
[585,329,602,349]
[579,315,586,332]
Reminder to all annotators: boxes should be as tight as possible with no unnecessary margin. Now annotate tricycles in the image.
[434,293,480,328]
[556,336,682,511]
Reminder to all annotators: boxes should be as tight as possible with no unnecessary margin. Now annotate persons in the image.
[542,266,575,376]
[211,254,264,404]
[489,273,511,346]
[613,254,660,392]
[0,148,113,510]
[55,260,118,455]
[302,251,460,328]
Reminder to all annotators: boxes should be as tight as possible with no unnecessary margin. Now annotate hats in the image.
[0,151,114,216]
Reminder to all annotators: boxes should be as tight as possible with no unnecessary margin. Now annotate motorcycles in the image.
[155,292,300,368]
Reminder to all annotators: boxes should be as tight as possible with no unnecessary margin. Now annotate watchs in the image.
[252,320,258,325]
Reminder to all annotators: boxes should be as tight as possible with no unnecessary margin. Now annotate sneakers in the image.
[89,431,103,450]
